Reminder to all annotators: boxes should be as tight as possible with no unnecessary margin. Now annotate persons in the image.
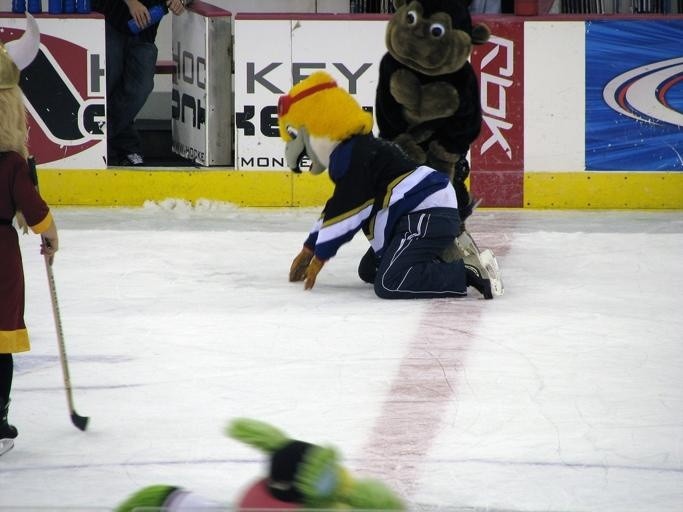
[91,0,191,167]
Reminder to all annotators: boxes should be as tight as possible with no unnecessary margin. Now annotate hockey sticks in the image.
[39,237,104,432]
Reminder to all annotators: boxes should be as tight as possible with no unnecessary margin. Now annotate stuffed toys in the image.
[0,50,58,439]
[110,418,408,512]
[277,71,492,300]
[376,0,489,220]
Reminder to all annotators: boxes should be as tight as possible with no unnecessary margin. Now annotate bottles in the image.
[127,4,170,34]
[12,0,91,15]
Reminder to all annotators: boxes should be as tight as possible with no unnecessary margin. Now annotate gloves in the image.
[303,256,324,291]
[290,246,312,280]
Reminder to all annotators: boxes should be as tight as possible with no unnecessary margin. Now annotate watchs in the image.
[180,0,184,6]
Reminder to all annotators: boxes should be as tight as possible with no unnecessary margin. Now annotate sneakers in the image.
[122,154,145,166]
[436,238,469,263]
[463,255,493,300]
